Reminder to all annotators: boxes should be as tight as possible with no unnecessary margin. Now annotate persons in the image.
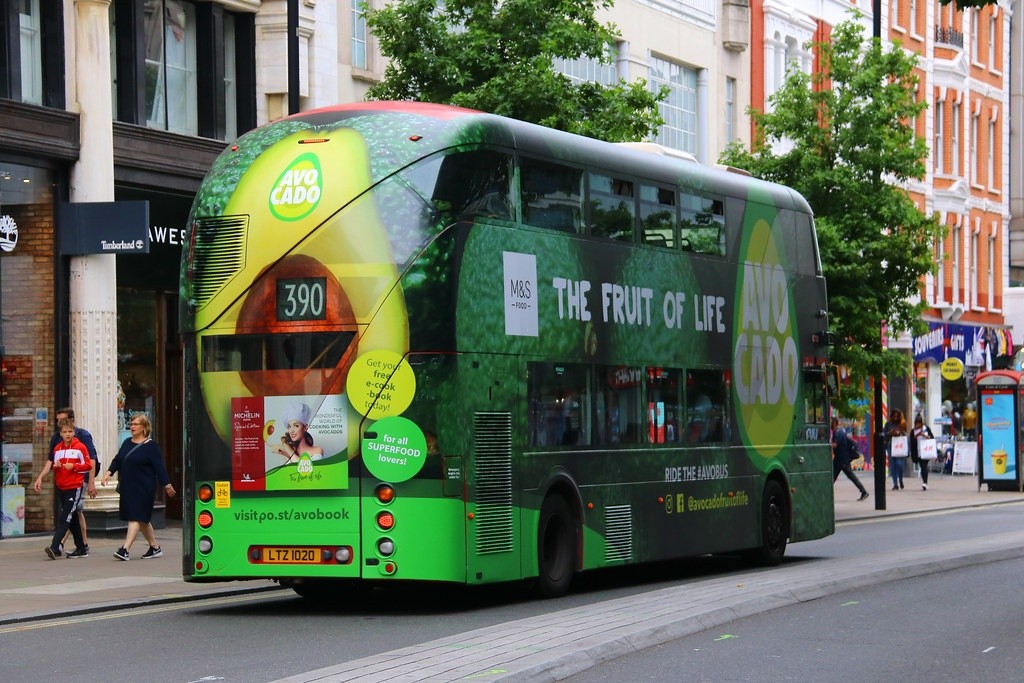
[883,408,908,489]
[32,407,98,558]
[100,414,175,560]
[269,402,323,462]
[830,417,870,501]
[910,412,934,489]
[942,400,977,441]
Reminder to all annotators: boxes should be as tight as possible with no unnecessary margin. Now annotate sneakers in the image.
[114,546,129,560]
[141,545,162,559]
[67,551,88,558]
[45,547,57,559]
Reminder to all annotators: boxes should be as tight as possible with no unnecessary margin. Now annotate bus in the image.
[178,100,842,601]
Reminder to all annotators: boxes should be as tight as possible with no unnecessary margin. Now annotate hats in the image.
[284,403,311,427]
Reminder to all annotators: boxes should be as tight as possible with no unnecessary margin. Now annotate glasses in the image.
[128,422,144,427]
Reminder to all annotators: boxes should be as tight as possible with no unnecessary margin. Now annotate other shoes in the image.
[899,479,904,489]
[58,544,63,552]
[858,492,869,501]
[892,486,899,490]
[84,544,89,551]
[922,483,927,490]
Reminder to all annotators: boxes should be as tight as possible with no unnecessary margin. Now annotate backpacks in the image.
[842,431,859,460]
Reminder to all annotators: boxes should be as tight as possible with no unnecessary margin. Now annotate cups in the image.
[990,454,1008,475]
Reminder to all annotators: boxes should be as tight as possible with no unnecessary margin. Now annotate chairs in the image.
[524,205,691,252]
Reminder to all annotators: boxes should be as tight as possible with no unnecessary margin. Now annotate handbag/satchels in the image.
[920,437,938,460]
[891,434,908,457]
[115,470,120,494]
